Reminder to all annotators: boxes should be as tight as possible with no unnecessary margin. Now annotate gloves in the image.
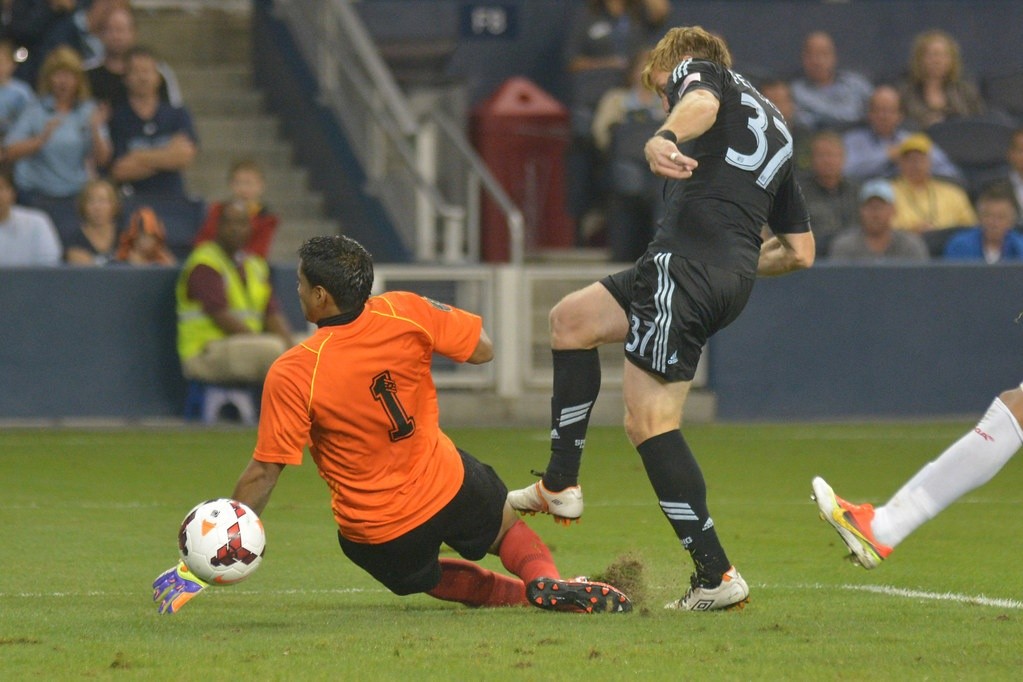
[152,559,210,616]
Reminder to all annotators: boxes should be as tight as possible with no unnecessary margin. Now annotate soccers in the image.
[176,497,267,588]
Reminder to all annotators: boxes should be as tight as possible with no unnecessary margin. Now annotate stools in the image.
[181,379,257,427]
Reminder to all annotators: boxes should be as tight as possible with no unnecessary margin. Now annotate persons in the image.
[546,0,1023,262]
[0,0,278,265]
[507,26,815,611]
[812,382,1023,570]
[151,236,633,615]
[176,199,295,382]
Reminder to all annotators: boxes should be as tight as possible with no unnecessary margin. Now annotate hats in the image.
[899,131,933,156]
[858,178,895,206]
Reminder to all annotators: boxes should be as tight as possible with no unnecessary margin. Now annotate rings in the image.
[670,153,677,161]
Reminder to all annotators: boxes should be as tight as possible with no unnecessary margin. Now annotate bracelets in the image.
[654,130,677,143]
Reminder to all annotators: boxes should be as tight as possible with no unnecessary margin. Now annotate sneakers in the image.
[507,469,584,527]
[811,475,893,570]
[525,575,634,614]
[663,565,750,612]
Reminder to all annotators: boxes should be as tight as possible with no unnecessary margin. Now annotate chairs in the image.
[570,66,1023,265]
